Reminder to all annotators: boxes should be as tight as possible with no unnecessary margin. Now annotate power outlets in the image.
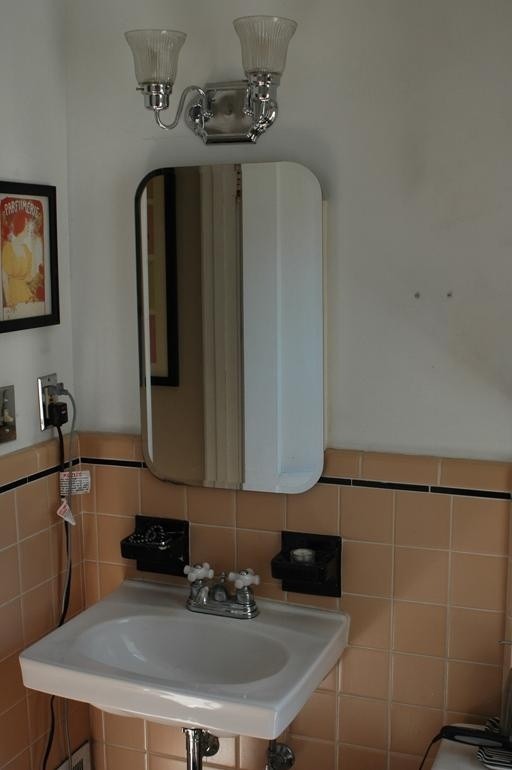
[0,385,16,445]
[38,373,60,432]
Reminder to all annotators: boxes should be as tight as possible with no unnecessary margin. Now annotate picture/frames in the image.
[0,179,61,334]
[142,167,181,387]
[57,741,94,770]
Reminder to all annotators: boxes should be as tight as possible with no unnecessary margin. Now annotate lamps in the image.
[125,16,298,146]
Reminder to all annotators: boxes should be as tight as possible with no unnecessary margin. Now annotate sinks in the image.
[19,578,349,741]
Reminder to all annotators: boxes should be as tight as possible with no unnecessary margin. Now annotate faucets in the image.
[183,561,261,620]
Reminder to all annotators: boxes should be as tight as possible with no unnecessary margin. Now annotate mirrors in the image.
[134,161,328,494]
